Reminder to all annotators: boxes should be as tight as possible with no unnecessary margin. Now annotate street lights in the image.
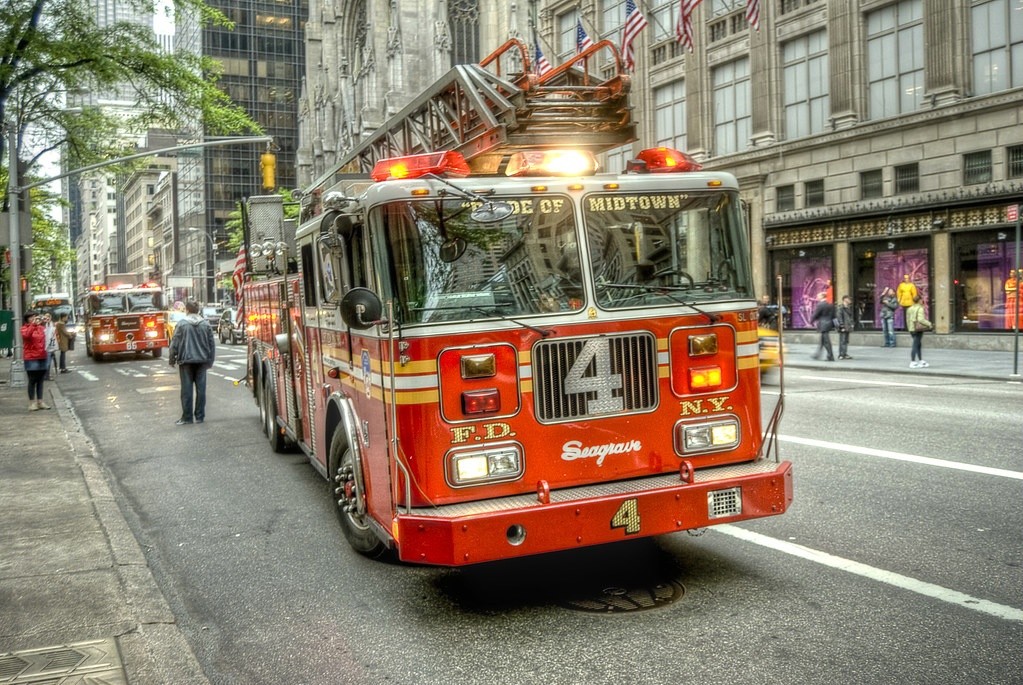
[188,227,218,303]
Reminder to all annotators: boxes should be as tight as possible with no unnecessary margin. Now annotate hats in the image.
[842,295,853,301]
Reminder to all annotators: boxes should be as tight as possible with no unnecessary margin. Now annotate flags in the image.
[577,18,594,66]
[621,0,648,74]
[746,0,760,39]
[675,0,702,55]
[534,40,553,76]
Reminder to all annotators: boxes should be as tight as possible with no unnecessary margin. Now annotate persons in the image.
[905,296,933,369]
[825,279,835,328]
[879,287,897,347]
[897,275,919,330]
[169,301,214,425]
[1005,269,1023,331]
[18,309,77,410]
[857,302,865,328]
[809,293,836,361]
[757,295,775,330]
[837,295,859,360]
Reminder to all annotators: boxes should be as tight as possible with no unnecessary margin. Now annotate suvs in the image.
[217,308,249,345]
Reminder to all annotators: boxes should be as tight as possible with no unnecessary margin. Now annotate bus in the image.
[31,293,77,351]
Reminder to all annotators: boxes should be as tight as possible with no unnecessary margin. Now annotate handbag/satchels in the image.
[914,307,929,332]
[44,332,59,353]
[68,333,75,350]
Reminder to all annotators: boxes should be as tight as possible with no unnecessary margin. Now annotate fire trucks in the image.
[83,272,171,362]
[239,37,795,567]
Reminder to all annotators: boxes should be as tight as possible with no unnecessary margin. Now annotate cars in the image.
[199,303,227,334]
[758,325,786,377]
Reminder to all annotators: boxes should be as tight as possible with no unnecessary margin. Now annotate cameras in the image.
[41,317,48,322]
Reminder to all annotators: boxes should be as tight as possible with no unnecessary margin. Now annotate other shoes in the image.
[825,359,834,361]
[44,376,55,381]
[174,418,193,425]
[891,343,896,347]
[844,355,852,359]
[37,403,51,409]
[881,343,890,347]
[910,361,921,368]
[810,355,819,360]
[61,369,72,374]
[918,360,929,368]
[195,417,203,423]
[27,403,38,411]
[838,356,844,360]
